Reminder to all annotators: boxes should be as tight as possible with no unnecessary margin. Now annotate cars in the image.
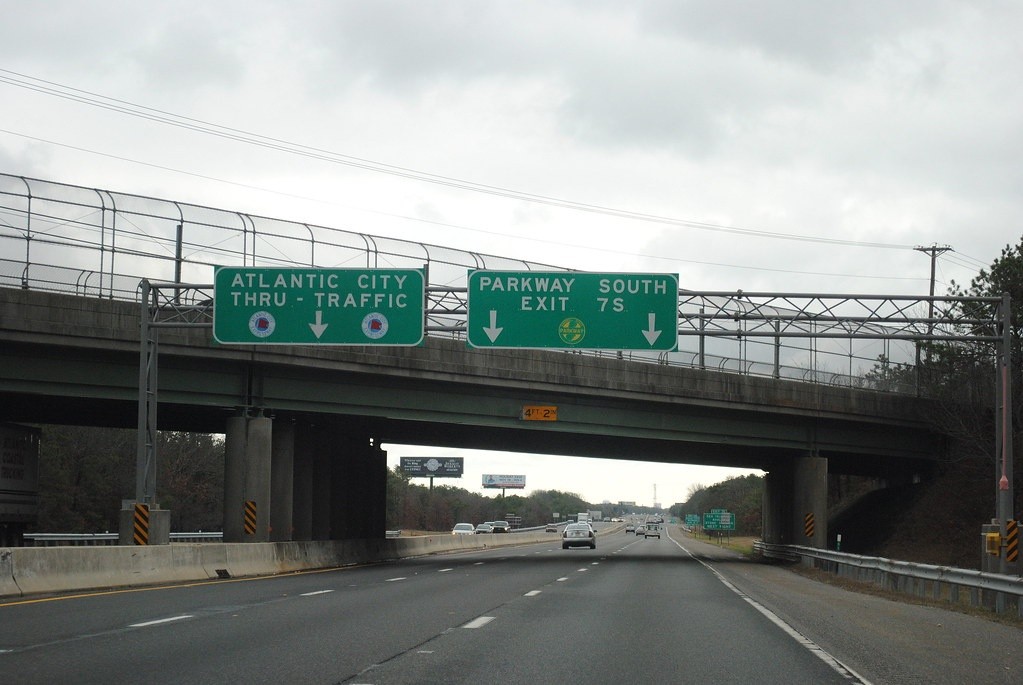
[484,522,494,529]
[493,521,511,533]
[475,524,492,534]
[645,515,664,524]
[566,513,626,527]
[450,523,476,535]
[546,524,557,533]
[644,524,663,540]
[562,523,598,550]
[636,525,646,536]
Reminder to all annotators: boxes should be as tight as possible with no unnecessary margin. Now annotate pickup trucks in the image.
[626,524,635,534]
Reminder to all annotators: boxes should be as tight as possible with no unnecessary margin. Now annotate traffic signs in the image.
[466,269,679,353]
[214,264,425,346]
